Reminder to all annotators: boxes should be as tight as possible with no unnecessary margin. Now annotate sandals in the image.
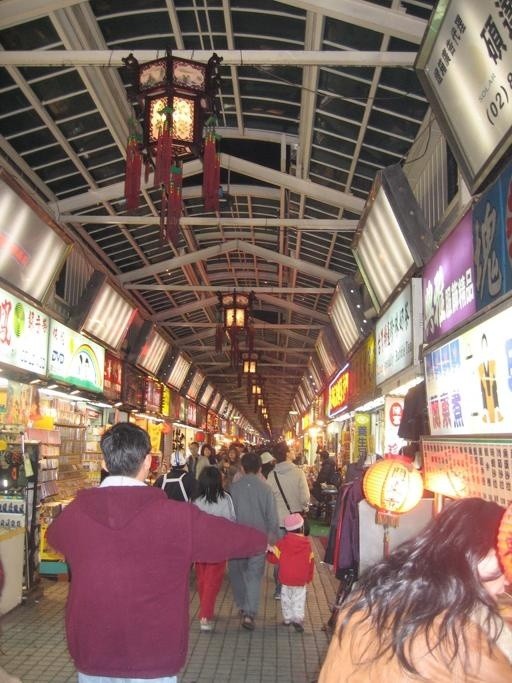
[239,612,256,632]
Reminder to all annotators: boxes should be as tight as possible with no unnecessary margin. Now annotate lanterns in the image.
[214,287,281,451]
[362,452,427,527]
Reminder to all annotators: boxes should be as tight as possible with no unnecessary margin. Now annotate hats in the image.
[284,512,305,532]
[170,450,186,467]
[259,451,275,465]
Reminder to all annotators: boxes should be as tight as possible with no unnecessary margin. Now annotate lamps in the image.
[235,348,275,440]
[215,289,255,348]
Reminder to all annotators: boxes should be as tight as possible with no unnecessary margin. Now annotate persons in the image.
[45,422,279,683]
[319,497,512,682]
[315,449,341,515]
[154,432,313,634]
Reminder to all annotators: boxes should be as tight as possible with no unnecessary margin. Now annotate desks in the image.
[319,484,339,526]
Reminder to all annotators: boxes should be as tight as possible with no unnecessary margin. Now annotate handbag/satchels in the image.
[303,513,311,537]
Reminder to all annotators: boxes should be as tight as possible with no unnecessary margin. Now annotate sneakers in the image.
[199,618,215,632]
[283,616,304,633]
[272,592,281,600]
